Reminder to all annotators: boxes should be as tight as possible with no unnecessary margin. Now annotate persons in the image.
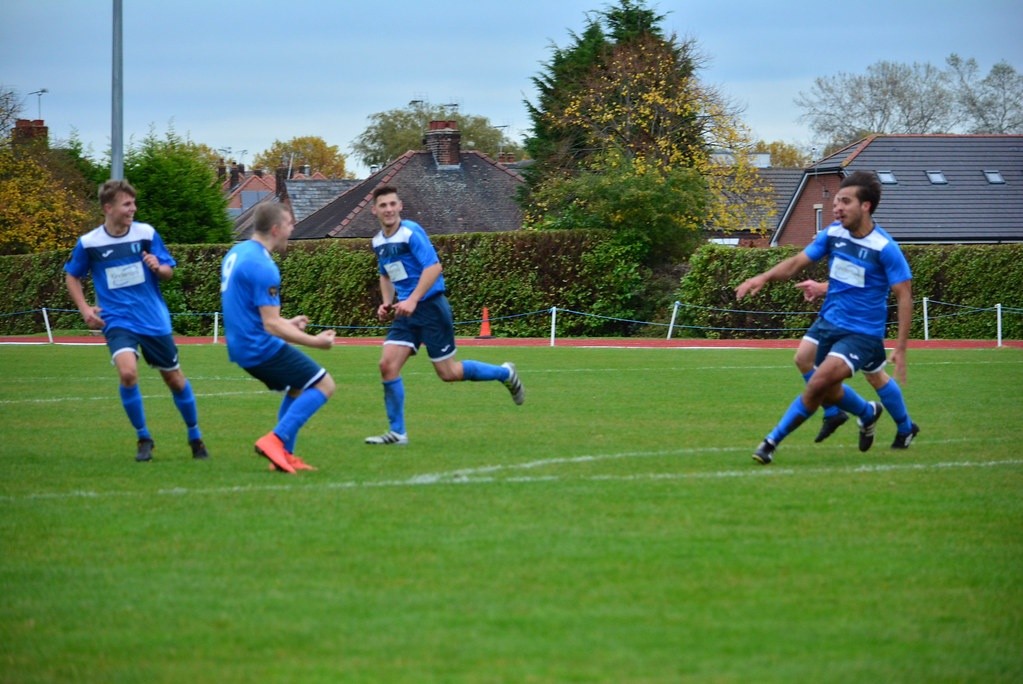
[219,201,336,475]
[60,178,210,460]
[365,186,525,445]
[733,172,914,464]
[793,192,919,450]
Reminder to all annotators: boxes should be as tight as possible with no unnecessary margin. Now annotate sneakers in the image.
[267,450,319,472]
[134,438,155,463]
[856,400,883,452]
[188,438,208,459]
[500,361,525,406]
[252,429,298,474]
[751,438,774,464]
[890,423,920,449]
[363,430,408,446]
[815,409,849,443]
